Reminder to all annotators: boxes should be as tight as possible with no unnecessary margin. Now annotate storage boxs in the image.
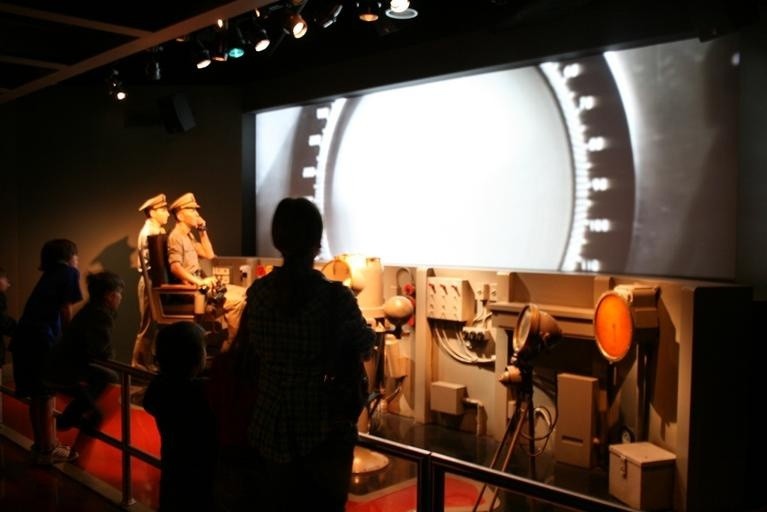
[608,442,678,510]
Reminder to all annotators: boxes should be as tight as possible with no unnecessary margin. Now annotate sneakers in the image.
[28,444,80,464]
[130,361,161,374]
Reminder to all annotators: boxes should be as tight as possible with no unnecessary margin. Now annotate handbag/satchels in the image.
[328,281,370,425]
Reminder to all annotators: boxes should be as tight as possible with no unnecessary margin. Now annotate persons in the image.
[141,322,221,512]
[0,267,20,369]
[55,272,125,468]
[234,198,377,512]
[11,238,83,465]
[166,192,218,287]
[130,192,171,372]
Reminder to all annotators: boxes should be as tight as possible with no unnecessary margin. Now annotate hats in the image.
[169,191,201,212]
[137,193,167,212]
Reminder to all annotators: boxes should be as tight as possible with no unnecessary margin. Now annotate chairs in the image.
[138,233,211,326]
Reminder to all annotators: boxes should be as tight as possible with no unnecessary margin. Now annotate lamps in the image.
[97,0,423,104]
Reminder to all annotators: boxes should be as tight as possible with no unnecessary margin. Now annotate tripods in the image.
[473,388,536,512]
